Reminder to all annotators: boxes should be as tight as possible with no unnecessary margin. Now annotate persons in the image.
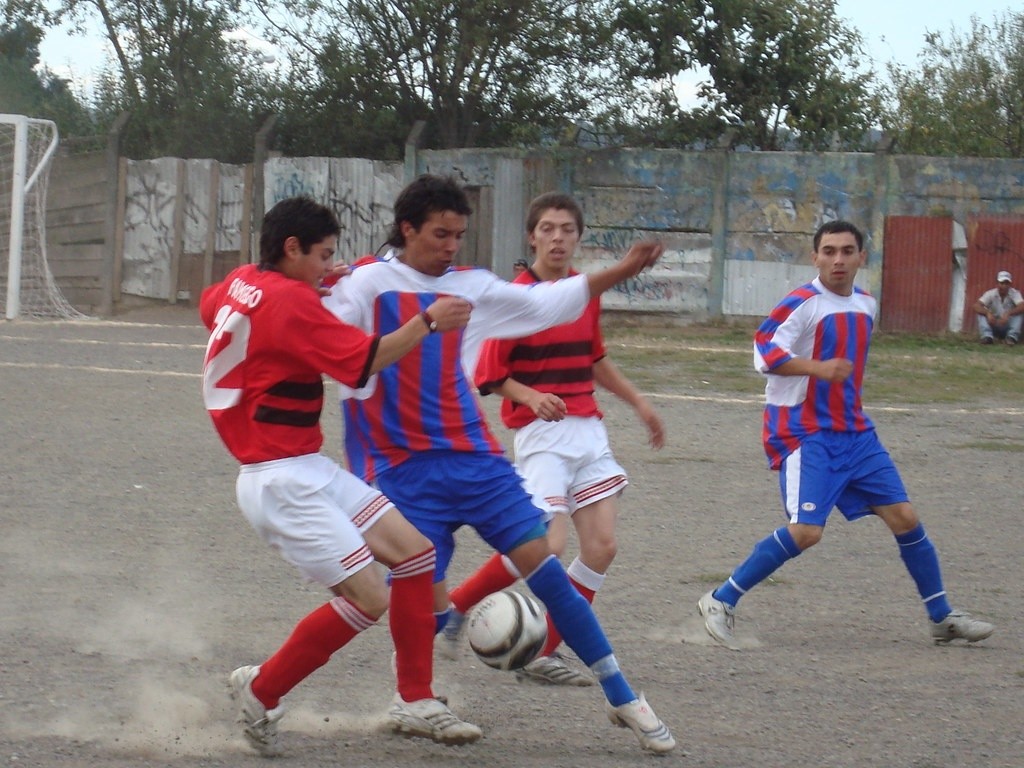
[322,171,677,755]
[442,192,668,686]
[696,220,996,648]
[200,197,483,757]
[972,270,1024,346]
[513,258,528,277]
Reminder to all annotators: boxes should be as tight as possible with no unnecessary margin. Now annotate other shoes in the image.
[980,337,993,344]
[1004,338,1016,345]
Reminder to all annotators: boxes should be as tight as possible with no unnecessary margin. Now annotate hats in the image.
[997,270,1013,283]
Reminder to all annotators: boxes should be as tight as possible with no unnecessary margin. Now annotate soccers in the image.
[464,590,550,673]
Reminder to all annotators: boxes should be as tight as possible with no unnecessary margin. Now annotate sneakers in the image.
[435,591,464,660]
[696,588,735,644]
[928,609,995,642]
[226,665,285,756]
[385,691,483,743]
[515,651,594,686]
[605,693,676,753]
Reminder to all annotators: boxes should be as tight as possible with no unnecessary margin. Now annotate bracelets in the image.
[419,309,438,333]
